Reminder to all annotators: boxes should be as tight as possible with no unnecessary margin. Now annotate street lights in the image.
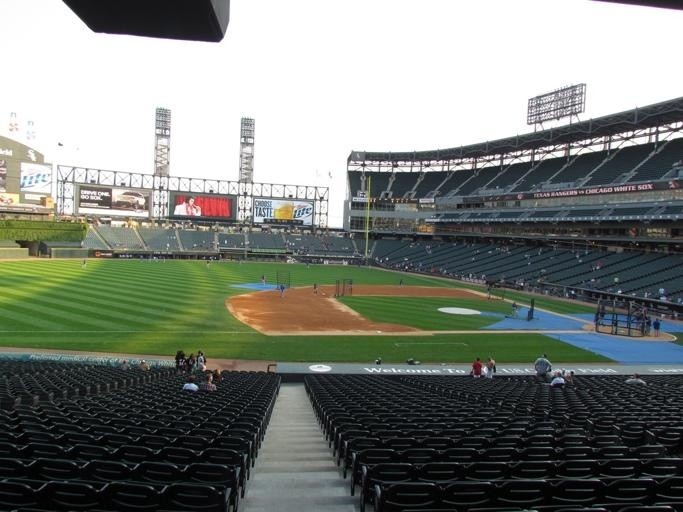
[9,111,18,139]
[26,120,36,148]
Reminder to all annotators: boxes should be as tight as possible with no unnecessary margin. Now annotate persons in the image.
[175,196,202,217]
[653,318,660,337]
[137,360,151,371]
[534,354,574,389]
[137,228,683,319]
[175,349,224,392]
[626,376,646,387]
[121,361,132,371]
[645,316,651,336]
[486,358,496,379]
[470,360,483,378]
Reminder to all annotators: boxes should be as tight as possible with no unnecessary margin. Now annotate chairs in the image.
[1,351,281,511]
[305,372,683,510]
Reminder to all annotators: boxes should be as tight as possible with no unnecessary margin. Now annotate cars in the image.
[115,191,146,209]
